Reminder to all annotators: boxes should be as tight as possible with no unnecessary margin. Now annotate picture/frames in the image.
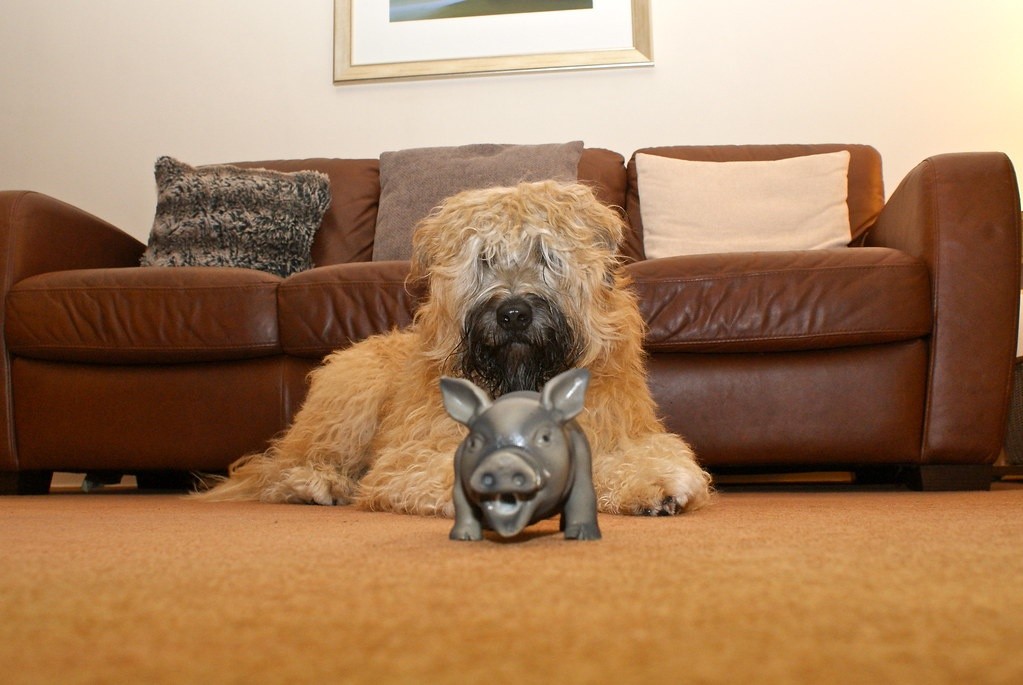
[333,0,654,83]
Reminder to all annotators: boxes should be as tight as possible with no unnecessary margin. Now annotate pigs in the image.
[439,364,603,543]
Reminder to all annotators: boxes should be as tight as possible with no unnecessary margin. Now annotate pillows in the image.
[635,149,852,258]
[371,140,586,260]
[139,155,331,273]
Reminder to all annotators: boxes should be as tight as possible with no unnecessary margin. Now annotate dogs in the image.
[178,180,714,518]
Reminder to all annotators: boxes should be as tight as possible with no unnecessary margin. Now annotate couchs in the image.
[0,143,1022,490]
